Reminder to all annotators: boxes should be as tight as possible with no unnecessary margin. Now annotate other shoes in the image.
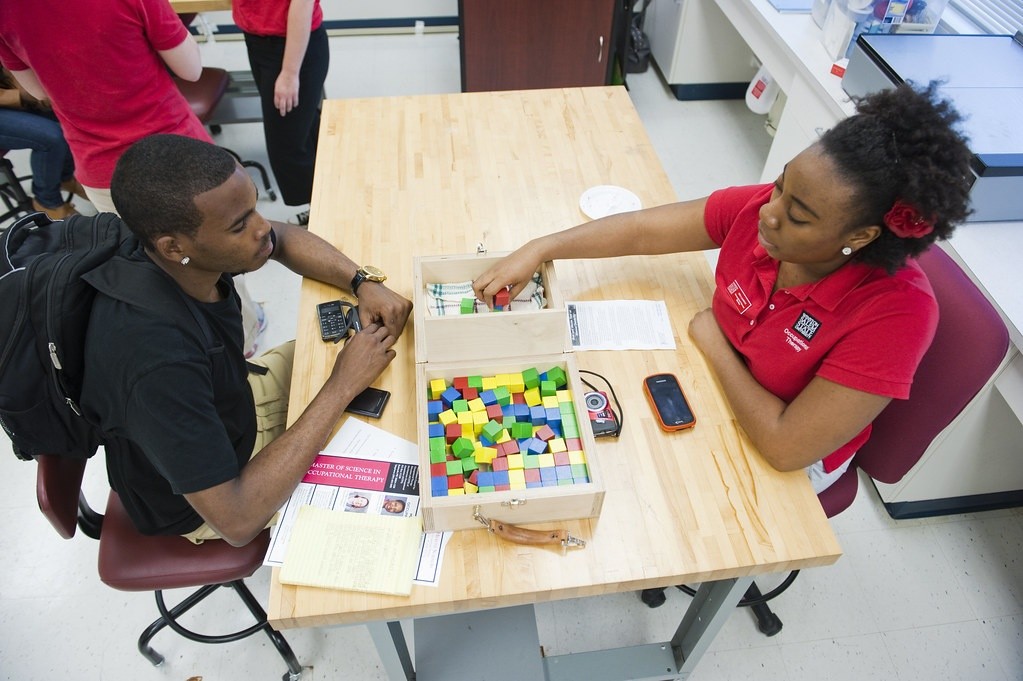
[32,200,79,220]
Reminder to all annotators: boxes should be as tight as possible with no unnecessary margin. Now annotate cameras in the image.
[583,391,617,438]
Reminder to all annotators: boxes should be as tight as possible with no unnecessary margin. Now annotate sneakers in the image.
[287,208,310,229]
[244,301,266,359]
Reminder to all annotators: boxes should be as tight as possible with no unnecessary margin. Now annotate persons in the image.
[347,495,368,511]
[472,76,979,493]
[1,0,268,357]
[79,134,415,548]
[231,0,330,228]
[383,498,406,513]
[0,65,90,221]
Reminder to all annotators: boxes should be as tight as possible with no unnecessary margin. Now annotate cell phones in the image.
[316,300,349,343]
[344,387,391,419]
[643,373,697,433]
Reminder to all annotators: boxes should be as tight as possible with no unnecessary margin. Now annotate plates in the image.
[580,185,642,220]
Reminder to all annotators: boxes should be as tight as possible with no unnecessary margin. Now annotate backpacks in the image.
[0,213,242,459]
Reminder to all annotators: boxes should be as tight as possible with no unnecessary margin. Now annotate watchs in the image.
[350,265,387,299]
